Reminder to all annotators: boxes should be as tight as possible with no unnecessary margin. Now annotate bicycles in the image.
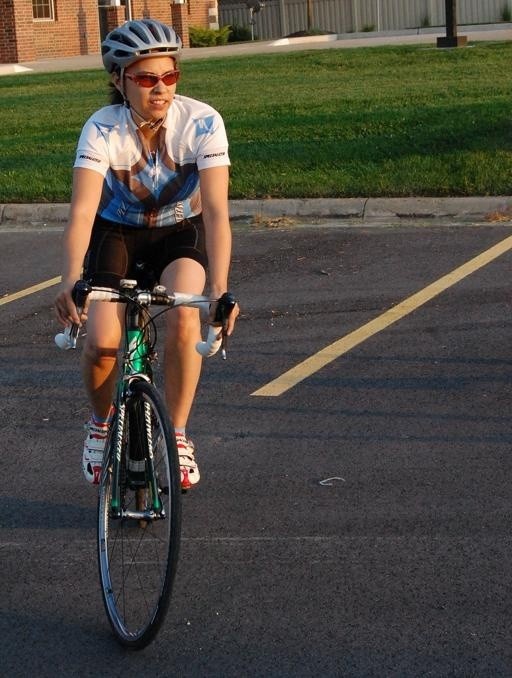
[53,276,240,652]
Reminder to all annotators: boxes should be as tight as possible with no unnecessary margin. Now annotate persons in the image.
[56,19,240,489]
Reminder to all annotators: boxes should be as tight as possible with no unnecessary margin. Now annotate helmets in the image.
[101,19,183,73]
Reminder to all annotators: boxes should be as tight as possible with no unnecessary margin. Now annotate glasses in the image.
[123,67,180,88]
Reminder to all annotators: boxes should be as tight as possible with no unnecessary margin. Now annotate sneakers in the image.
[82,406,115,485]
[174,432,201,490]
